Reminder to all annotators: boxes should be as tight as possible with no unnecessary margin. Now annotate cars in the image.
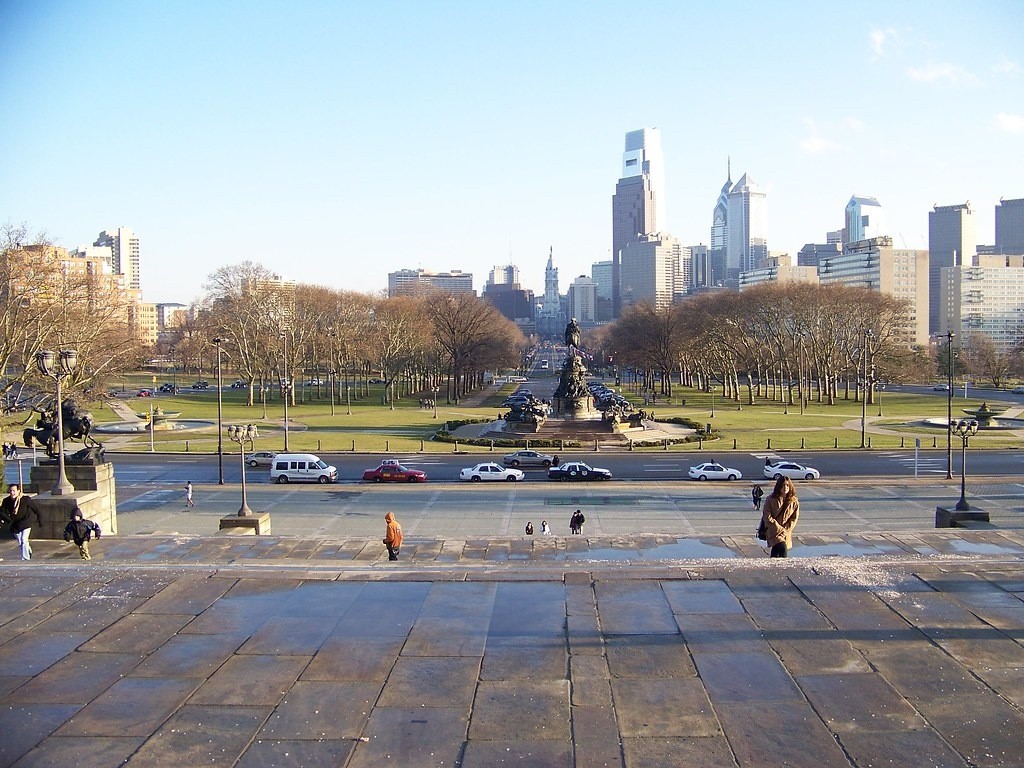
[362,449,613,484]
[933,384,1024,394]
[689,462,821,480]
[7,379,396,400]
[245,451,278,466]
[502,380,628,419]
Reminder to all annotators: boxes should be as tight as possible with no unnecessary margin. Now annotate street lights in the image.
[347,386,351,415]
[949,419,980,510]
[214,337,230,484]
[936,334,953,480]
[328,368,336,417]
[280,334,288,452]
[709,386,717,416]
[796,332,807,413]
[431,387,439,418]
[37,349,79,495]
[738,385,742,410]
[858,331,875,447]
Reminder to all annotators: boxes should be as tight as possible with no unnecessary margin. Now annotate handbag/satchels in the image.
[754,516,766,541]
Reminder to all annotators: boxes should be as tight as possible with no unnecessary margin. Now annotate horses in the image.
[23,396,104,460]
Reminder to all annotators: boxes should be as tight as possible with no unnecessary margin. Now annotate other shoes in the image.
[191,504,195,508]
[186,505,189,507]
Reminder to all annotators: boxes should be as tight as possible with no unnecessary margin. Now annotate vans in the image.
[271,454,339,483]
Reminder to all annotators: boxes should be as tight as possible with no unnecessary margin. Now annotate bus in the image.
[542,360,549,368]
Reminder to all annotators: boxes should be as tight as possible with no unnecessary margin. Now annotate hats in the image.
[577,510,581,513]
[188,481,191,484]
[69,508,83,519]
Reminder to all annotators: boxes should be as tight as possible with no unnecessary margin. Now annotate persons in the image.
[766,457,771,465]
[569,509,585,535]
[762,476,799,557]
[526,522,533,535]
[383,511,403,561]
[31,401,58,444]
[751,485,764,510]
[565,317,581,356]
[539,520,550,535]
[419,399,435,410]
[64,508,101,560]
[0,484,43,560]
[3,441,18,459]
[184,481,195,507]
[552,455,559,467]
